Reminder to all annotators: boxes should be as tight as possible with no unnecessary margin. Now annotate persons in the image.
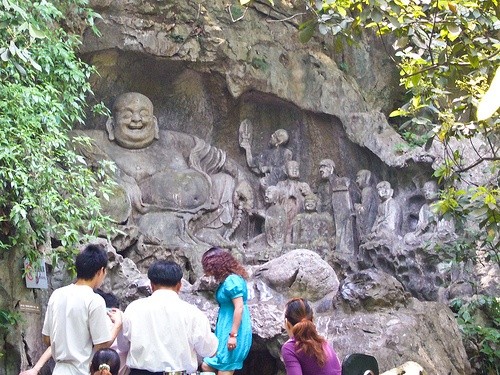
[66,92,253,246]
[201,247,252,374]
[281,298,341,375]
[318,158,361,256]
[289,193,335,247]
[18,289,123,375]
[89,348,121,375]
[253,186,289,245]
[367,180,403,239]
[241,128,294,174]
[354,169,380,235]
[276,160,319,216]
[404,181,455,245]
[41,244,123,375]
[122,259,218,375]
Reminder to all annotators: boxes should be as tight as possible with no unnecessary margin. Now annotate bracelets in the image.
[228,333,239,338]
